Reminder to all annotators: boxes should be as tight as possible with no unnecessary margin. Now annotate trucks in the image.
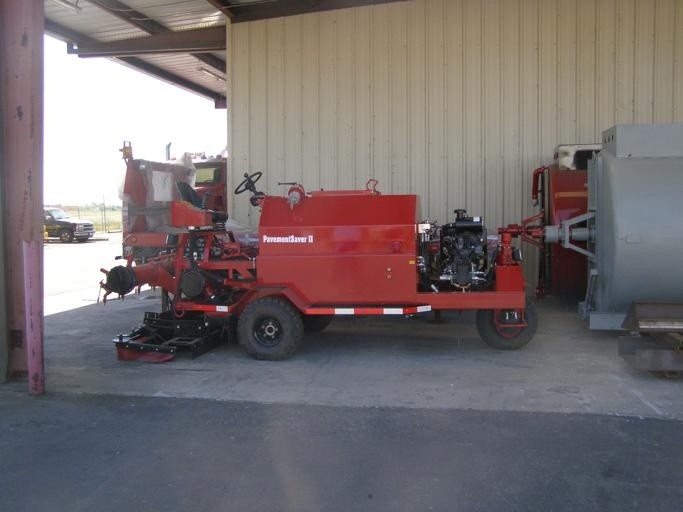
[159,142,228,221]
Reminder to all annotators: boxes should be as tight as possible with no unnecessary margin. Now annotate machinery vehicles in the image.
[99,140,540,365]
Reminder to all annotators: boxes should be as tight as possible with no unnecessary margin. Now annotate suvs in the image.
[44,208,95,243]
